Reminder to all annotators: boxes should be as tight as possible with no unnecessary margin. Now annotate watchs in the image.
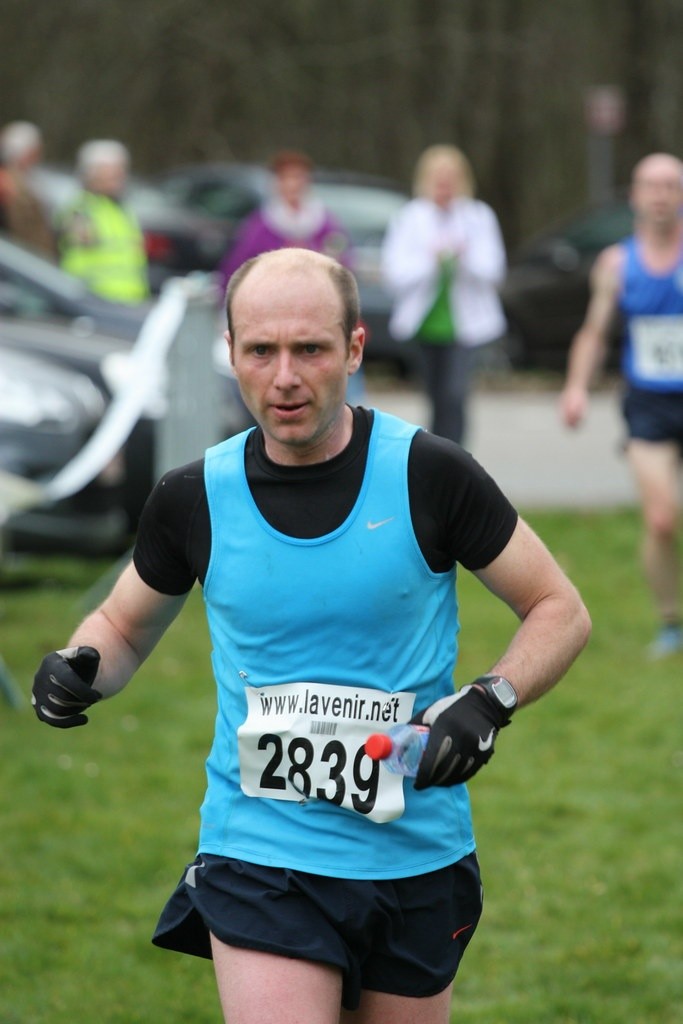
[473,676,518,728]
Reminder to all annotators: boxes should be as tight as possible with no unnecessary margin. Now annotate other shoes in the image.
[650,622,683,661]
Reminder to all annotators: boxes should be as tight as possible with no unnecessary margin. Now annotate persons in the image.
[48,141,157,530]
[1,122,52,260]
[381,146,507,446]
[33,248,591,1024]
[213,151,363,431]
[563,155,682,659]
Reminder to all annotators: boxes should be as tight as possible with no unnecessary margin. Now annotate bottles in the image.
[364,725,430,778]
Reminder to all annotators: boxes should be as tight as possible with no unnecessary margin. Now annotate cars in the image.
[161,162,424,358]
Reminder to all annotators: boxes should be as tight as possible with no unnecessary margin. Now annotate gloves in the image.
[31,644,102,727]
[397,682,511,790]
